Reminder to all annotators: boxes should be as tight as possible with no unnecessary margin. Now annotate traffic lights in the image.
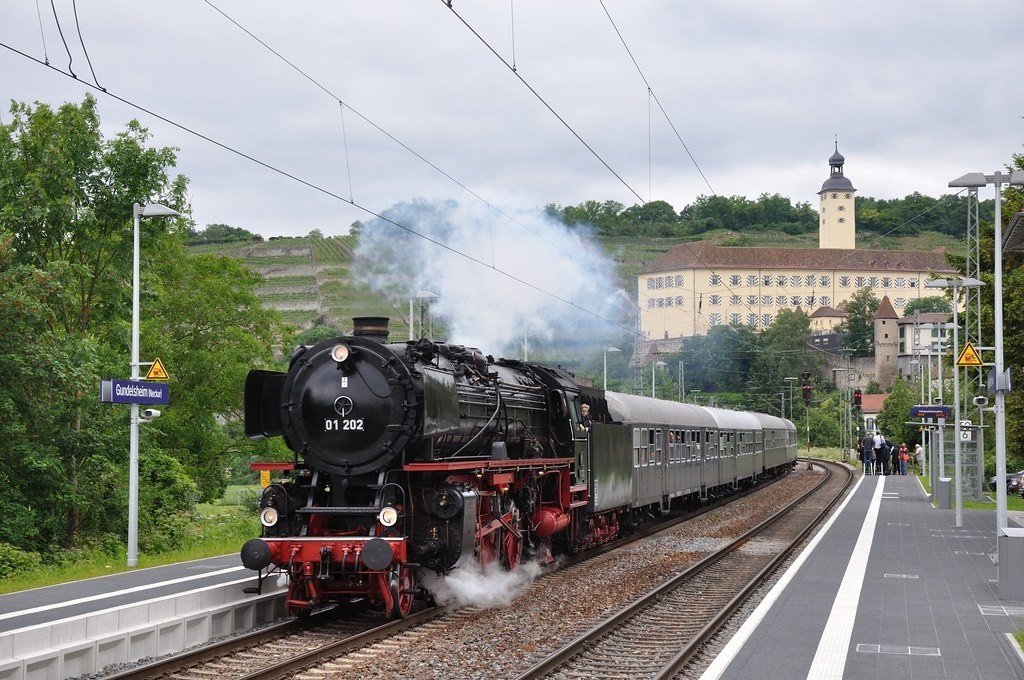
[801,372,811,406]
[855,390,861,410]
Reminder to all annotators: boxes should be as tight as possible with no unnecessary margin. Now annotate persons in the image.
[898,443,909,476]
[913,444,926,475]
[579,404,590,432]
[891,445,900,475]
[857,443,864,472]
[881,442,890,476]
[861,432,873,467]
[873,431,886,467]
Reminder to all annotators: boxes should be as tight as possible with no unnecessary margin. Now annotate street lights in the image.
[917,321,963,480]
[117,203,180,567]
[652,360,668,399]
[524,323,544,360]
[603,346,621,390]
[949,169,1024,528]
[924,277,986,527]
[912,344,946,427]
[408,288,440,341]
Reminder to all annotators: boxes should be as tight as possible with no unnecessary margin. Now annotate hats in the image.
[915,444,921,448]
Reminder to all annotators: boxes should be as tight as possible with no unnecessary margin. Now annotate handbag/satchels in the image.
[881,443,885,448]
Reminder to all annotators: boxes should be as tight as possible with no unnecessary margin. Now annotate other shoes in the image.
[920,473,923,475]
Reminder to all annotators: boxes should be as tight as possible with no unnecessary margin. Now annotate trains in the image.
[240,316,797,620]
